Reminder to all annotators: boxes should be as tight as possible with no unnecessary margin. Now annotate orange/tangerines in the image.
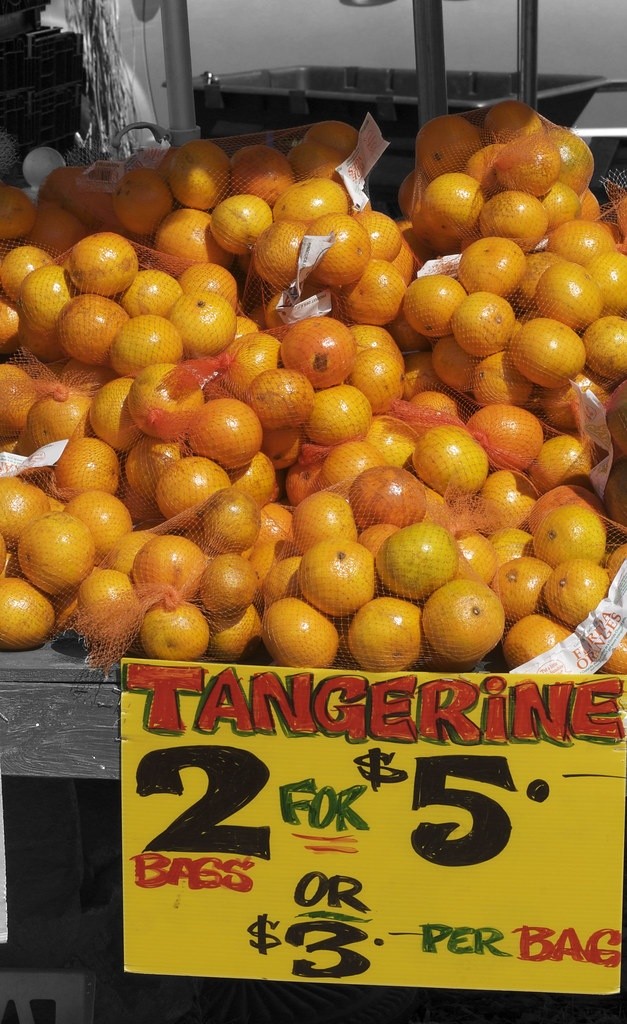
[0,97,627,679]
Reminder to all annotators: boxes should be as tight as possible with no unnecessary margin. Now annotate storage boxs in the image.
[187,64,607,137]
